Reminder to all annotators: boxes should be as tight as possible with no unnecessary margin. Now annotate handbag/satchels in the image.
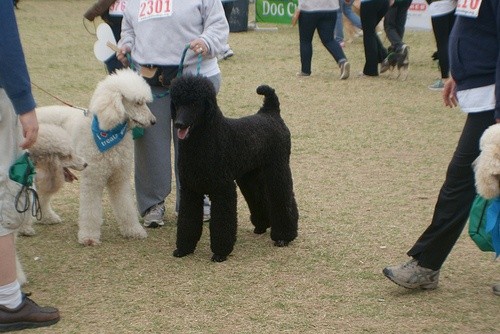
[468,193,495,252]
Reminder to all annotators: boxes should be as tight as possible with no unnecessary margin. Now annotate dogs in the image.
[169,74,300,262]
[381,44,411,80]
[23,66,157,246]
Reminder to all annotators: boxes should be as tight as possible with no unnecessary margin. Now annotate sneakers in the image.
[382,258,440,292]
[144,202,165,228]
[203,194,211,222]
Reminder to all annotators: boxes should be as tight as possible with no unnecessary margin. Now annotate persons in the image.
[383,0,500,296]
[116,0,229,228]
[426,0,457,91]
[0,0,61,332]
[359,0,389,77]
[334,0,362,46]
[384,0,413,71]
[292,0,350,80]
[100,0,131,74]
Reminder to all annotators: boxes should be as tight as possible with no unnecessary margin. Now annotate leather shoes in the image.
[0,292,60,333]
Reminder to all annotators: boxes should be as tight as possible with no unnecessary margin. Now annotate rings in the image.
[200,47,205,52]
[196,44,200,48]
[116,54,120,57]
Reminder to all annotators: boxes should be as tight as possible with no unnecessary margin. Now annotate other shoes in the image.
[401,44,408,55]
[339,62,350,80]
[428,79,444,90]
[358,72,379,79]
[297,72,310,76]
[380,57,389,73]
[218,42,234,60]
[340,41,345,47]
